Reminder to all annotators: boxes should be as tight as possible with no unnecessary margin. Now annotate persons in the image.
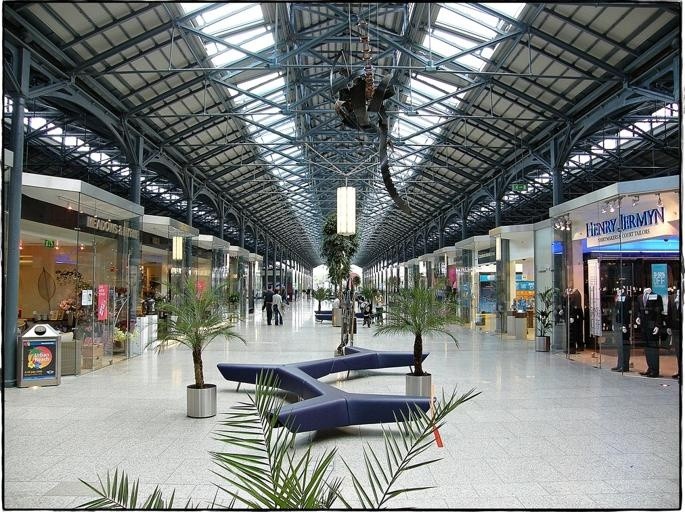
[559,288,682,379]
[353,290,384,328]
[263,285,275,326]
[266,288,285,326]
[281,286,289,305]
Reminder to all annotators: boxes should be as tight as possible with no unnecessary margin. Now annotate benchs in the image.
[216,345,433,451]
[315,313,364,324]
[315,310,333,314]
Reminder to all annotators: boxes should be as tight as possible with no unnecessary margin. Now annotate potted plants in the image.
[371,267,463,397]
[313,287,328,321]
[531,287,556,352]
[318,213,359,329]
[146,270,246,420]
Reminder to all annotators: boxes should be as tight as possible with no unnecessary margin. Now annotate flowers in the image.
[58,266,102,346]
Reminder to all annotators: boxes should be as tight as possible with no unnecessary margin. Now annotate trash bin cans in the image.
[16,324,61,387]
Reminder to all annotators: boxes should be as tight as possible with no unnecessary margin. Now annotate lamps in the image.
[172,231,183,260]
[554,216,573,232]
[600,191,664,215]
[336,175,357,239]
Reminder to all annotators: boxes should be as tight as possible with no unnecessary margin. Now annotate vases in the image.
[80,344,103,370]
